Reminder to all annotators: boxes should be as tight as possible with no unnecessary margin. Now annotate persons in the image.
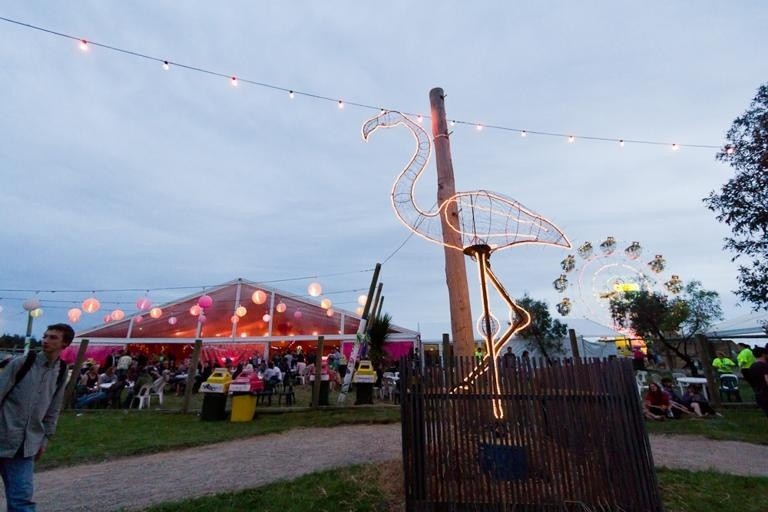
[616,344,644,371]
[746,346,768,419]
[639,400,666,422]
[519,350,533,378]
[503,347,517,381]
[661,378,697,417]
[0,323,76,512]
[735,343,761,408]
[644,383,676,419]
[475,347,483,359]
[711,350,743,401]
[67,345,425,409]
[681,382,723,418]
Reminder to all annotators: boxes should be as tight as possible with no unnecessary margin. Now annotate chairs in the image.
[256,367,311,406]
[128,375,167,410]
[379,371,400,401]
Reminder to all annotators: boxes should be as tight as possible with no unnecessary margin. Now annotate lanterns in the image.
[21,282,373,326]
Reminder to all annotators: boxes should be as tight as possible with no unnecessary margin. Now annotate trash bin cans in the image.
[198,368,264,422]
[352,360,378,405]
